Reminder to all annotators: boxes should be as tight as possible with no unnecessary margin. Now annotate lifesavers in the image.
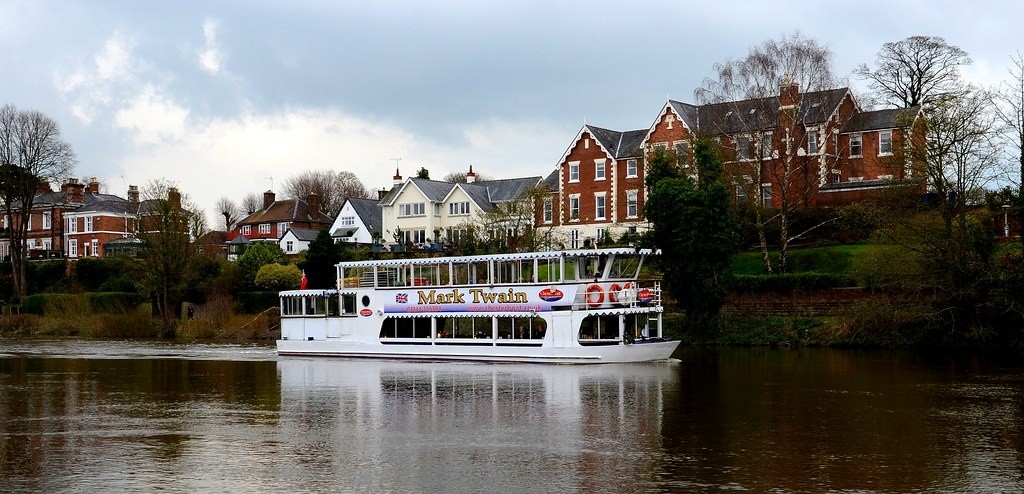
[609,283,623,307]
[584,285,604,307]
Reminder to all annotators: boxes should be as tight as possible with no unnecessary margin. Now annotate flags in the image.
[300,273,307,290]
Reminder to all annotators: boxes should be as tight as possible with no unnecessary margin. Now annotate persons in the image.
[438,325,547,339]
[187,304,194,320]
[440,267,618,285]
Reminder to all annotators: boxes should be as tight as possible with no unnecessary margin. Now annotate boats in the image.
[276,248,682,363]
